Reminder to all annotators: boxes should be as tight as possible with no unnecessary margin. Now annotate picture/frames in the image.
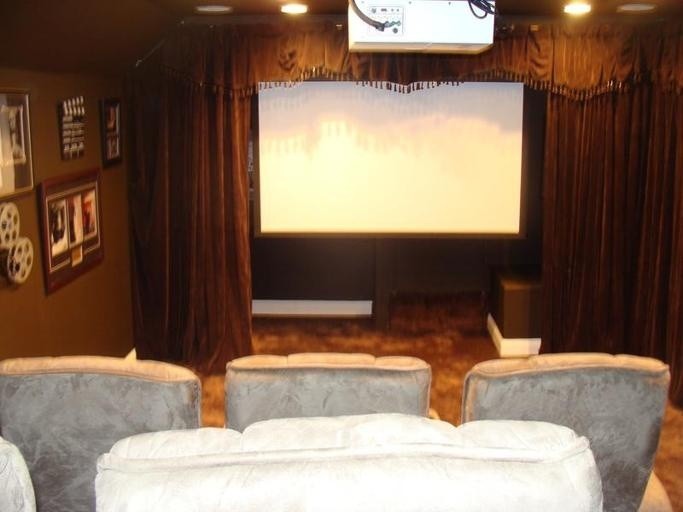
[0,88,36,203]
[39,165,105,295]
[99,96,125,166]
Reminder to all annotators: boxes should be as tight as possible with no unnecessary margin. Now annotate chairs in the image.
[0,355,202,512]
[223,353,432,430]
[457,353,671,512]
[94,411,604,511]
